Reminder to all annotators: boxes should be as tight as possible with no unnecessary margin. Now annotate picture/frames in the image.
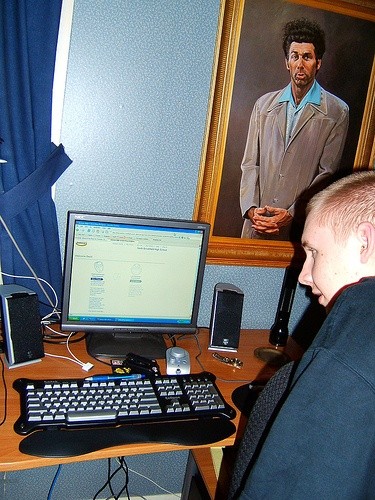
[193,0,375,269]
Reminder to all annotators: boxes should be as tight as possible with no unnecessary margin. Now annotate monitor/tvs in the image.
[61,210,210,358]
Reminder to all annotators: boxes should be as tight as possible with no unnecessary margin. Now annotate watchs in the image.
[213,353,243,369]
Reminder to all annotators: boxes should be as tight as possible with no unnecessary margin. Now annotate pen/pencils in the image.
[84,373,145,381]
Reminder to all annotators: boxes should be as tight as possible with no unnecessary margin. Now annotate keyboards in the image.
[11,371,238,436]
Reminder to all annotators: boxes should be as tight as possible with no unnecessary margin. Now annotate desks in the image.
[0,313,302,500]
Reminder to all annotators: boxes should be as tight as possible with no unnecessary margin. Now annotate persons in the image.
[230,171,375,500]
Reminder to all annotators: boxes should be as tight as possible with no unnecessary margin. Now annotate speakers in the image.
[0,284,45,365]
[208,282,244,353]
